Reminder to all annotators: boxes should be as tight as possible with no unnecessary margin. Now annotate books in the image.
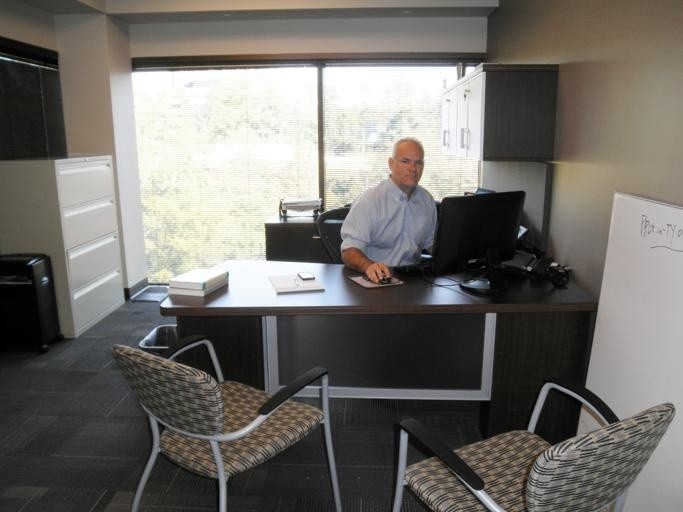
[169,268,229,295]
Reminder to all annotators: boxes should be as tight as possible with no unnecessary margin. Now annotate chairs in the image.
[315,205,351,264]
[109,333,343,511]
[392,380,677,512]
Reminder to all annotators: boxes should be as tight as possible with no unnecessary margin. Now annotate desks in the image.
[159,259,598,438]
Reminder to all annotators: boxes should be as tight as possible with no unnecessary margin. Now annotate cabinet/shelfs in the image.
[440,88,458,159]
[455,72,555,160]
[0,154,126,340]
[1,252,61,354]
[263,212,343,263]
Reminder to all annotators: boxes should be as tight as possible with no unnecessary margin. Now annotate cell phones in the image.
[297,271,316,280]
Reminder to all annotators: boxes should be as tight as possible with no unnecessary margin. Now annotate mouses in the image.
[378,275,393,285]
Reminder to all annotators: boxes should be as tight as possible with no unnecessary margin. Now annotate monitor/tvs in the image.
[434,190,526,302]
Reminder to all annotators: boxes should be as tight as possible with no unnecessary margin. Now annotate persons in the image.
[339,138,438,284]
